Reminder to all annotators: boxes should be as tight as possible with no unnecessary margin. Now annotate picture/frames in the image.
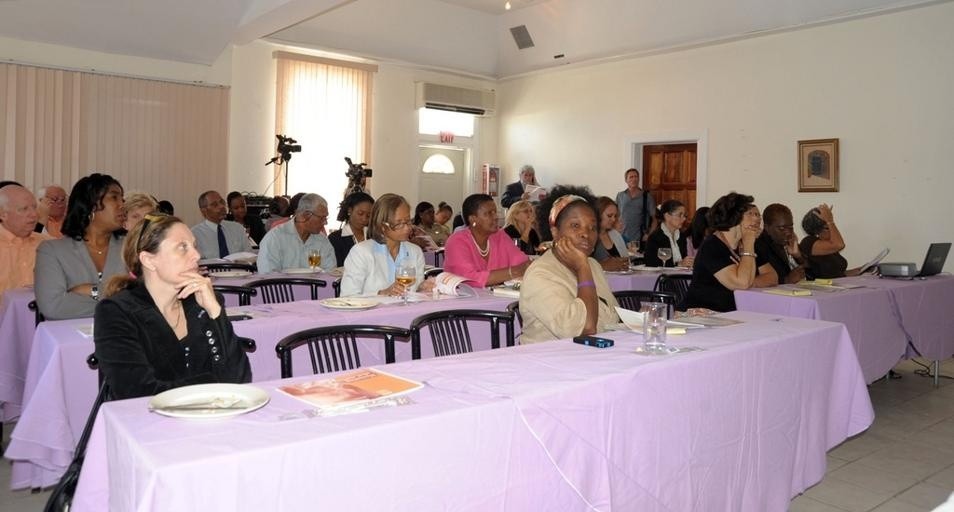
[795,137,841,195]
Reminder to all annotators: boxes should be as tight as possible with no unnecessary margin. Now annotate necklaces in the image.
[86,241,109,256]
[470,234,489,257]
[719,231,742,261]
[169,299,179,329]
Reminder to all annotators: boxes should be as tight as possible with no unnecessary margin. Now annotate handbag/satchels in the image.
[641,213,653,230]
[41,455,81,511]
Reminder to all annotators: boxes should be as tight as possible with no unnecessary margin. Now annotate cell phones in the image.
[573,335,613,348]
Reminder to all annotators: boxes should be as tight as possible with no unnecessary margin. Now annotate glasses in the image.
[305,210,328,222]
[670,210,688,218]
[382,218,415,230]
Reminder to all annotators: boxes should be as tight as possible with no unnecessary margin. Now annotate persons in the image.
[92,212,251,403]
[500,164,536,208]
[1,185,58,289]
[615,168,657,241]
[328,192,376,267]
[243,192,306,232]
[676,193,779,314]
[645,199,710,271]
[190,191,252,260]
[406,201,454,251]
[257,193,338,271]
[339,193,438,298]
[518,184,658,345]
[754,203,821,284]
[34,174,129,320]
[226,191,267,249]
[798,202,847,281]
[122,193,159,232]
[441,194,534,288]
[591,197,633,270]
[502,201,542,255]
[32,184,68,237]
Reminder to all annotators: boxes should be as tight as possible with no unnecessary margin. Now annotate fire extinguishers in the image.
[489,169,497,196]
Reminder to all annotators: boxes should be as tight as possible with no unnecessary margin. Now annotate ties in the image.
[215,224,229,259]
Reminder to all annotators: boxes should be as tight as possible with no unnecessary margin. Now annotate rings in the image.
[193,283,197,288]
[645,239,647,240]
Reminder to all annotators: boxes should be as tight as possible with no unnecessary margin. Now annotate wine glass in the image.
[396,262,415,309]
[657,247,672,271]
[308,248,322,277]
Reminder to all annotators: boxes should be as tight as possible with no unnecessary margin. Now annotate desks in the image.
[0,244,875,511]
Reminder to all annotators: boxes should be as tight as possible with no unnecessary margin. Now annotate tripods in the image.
[340,184,366,230]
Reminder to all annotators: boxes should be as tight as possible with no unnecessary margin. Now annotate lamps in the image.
[420,152,456,177]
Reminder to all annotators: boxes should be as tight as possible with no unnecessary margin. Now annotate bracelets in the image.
[825,222,834,225]
[739,251,758,258]
[508,265,515,279]
[576,281,600,289]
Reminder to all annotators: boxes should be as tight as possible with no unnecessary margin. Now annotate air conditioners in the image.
[411,81,499,121]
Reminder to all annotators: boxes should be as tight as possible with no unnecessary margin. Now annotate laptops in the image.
[914,243,952,276]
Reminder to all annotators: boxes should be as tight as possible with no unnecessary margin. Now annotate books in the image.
[425,272,481,301]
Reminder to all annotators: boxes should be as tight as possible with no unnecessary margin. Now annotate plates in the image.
[632,266,659,272]
[208,271,252,280]
[281,268,322,276]
[147,383,271,425]
[319,297,381,312]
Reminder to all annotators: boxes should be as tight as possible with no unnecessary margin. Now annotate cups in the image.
[512,237,522,250]
[628,239,640,252]
[641,301,669,355]
